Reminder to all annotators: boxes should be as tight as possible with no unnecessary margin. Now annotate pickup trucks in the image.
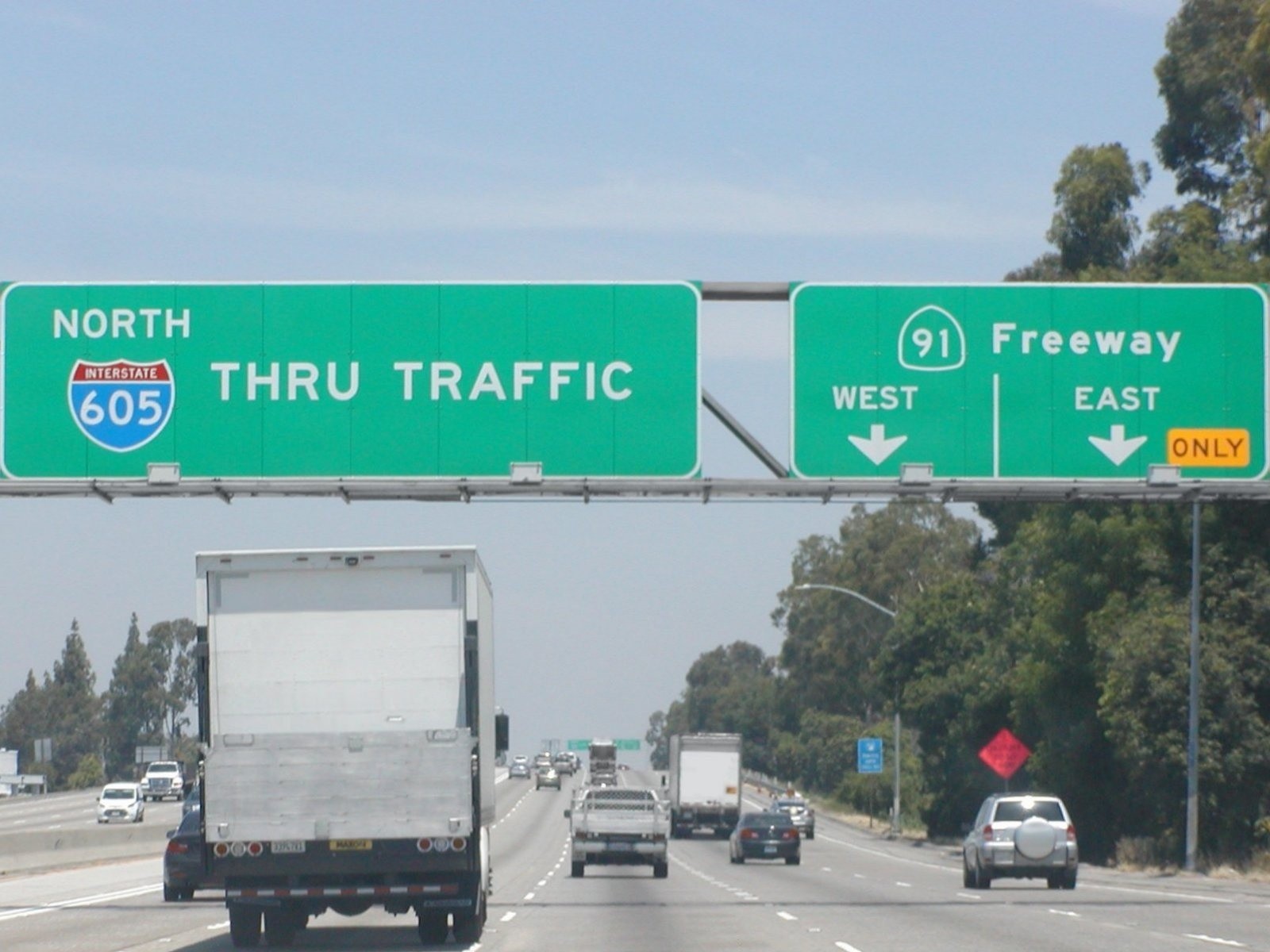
[564,787,671,878]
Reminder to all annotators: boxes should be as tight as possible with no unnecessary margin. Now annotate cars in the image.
[509,751,582,791]
[591,769,617,786]
[618,764,629,770]
[729,808,800,864]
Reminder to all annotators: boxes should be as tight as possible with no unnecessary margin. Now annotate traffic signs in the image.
[791,282,1267,482]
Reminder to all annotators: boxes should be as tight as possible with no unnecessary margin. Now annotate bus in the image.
[588,737,617,772]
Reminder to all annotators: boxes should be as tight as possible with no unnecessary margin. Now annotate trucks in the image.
[194,546,509,947]
[661,733,742,839]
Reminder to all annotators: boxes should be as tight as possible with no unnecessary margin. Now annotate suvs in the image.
[770,800,815,839]
[961,792,1078,889]
[96,760,225,902]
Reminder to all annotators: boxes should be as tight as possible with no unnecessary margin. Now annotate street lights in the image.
[795,584,899,838]
